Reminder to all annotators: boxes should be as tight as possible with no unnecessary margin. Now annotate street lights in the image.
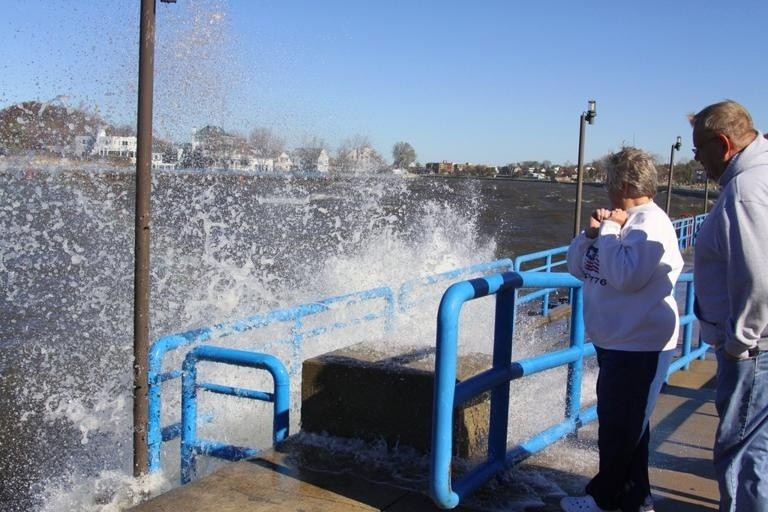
[574,99,596,237]
[666,135,681,217]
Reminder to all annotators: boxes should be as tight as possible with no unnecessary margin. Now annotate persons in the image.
[688,100,768,512]
[559,146,684,512]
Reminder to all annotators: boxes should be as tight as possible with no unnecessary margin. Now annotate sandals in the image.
[560,494,655,512]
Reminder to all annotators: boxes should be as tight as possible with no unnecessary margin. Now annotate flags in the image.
[583,246,600,273]
